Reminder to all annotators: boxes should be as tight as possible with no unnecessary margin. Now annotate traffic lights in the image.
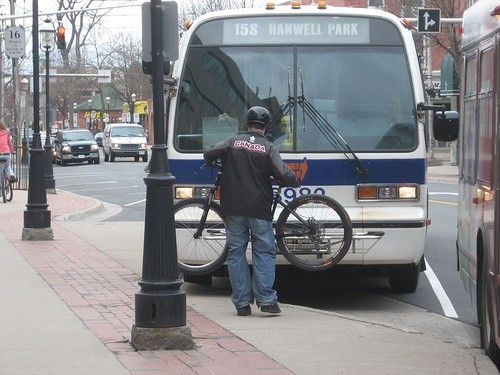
[57,27,65,50]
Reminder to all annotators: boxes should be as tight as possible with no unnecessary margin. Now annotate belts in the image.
[0,153,10,155]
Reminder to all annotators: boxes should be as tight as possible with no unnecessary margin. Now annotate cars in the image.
[20,128,58,164]
[94,132,103,146]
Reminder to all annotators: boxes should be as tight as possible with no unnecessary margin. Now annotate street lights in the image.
[37,19,57,193]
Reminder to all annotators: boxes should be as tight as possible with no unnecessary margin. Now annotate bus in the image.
[456,0,500,370]
[165,0,460,293]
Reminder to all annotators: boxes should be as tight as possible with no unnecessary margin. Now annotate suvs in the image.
[53,130,100,166]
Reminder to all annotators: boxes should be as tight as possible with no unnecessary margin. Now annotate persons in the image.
[203,106,301,316]
[0,121,17,196]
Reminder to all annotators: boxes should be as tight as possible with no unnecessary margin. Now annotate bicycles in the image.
[0,152,17,203]
[172,164,353,275]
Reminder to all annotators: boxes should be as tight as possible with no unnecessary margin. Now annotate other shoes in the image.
[10,175,18,182]
[261,301,282,313]
[236,304,252,316]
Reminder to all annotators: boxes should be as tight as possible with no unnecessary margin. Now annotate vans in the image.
[102,124,148,162]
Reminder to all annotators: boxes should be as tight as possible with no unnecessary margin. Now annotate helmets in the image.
[244,106,272,128]
